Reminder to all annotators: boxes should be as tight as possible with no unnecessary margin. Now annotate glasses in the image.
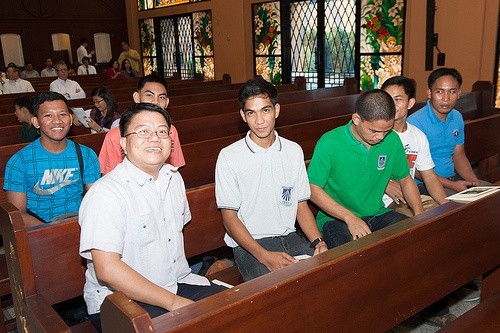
[92,99,103,105]
[127,130,171,139]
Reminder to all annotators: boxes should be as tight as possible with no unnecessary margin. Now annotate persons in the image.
[99,72,203,275]
[2,91,101,326]
[209,75,328,283]
[406,68,492,199]
[380,75,480,302]
[0,38,141,144]
[307,89,458,328]
[72,102,230,330]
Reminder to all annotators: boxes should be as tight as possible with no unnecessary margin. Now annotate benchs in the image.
[0,61,500,333]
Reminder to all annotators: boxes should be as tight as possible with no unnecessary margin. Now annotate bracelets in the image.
[309,236,326,247]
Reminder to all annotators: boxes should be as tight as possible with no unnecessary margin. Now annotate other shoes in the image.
[422,302,450,317]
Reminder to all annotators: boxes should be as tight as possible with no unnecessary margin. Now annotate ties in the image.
[87,69,89,74]
